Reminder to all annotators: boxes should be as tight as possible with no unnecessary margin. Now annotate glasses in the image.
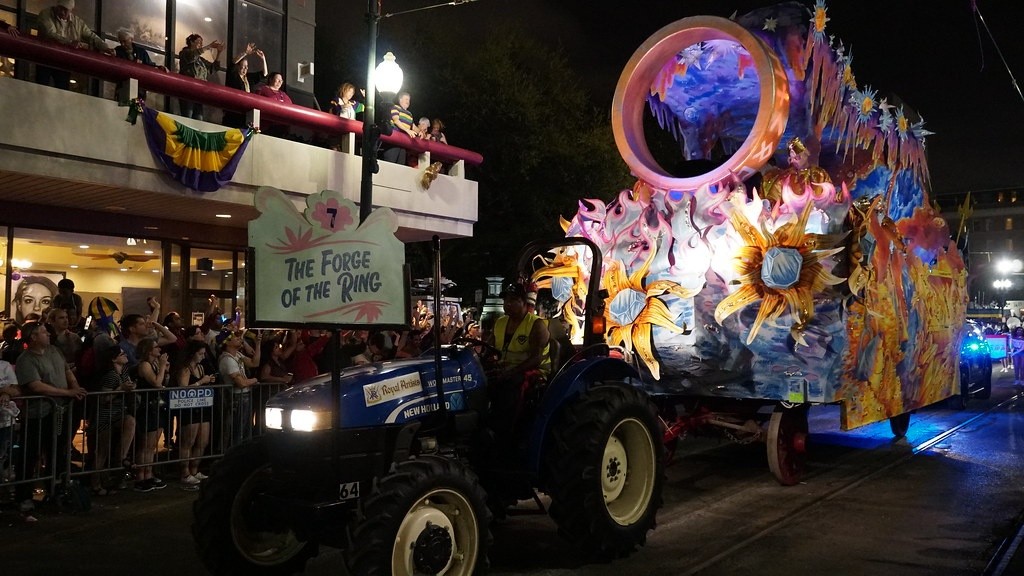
[153,344,160,349]
[116,353,128,358]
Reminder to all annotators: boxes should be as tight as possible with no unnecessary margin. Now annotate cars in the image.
[965,300,1024,364]
[944,319,994,410]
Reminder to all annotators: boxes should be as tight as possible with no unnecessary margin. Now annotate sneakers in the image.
[193,471,208,480]
[181,475,200,484]
[133,481,155,492]
[145,478,167,490]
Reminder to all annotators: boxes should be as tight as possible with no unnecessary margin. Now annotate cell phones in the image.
[257,330,260,335]
[213,373,221,377]
[286,373,293,383]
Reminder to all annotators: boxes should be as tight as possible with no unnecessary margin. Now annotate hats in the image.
[499,283,526,297]
[216,328,235,348]
[220,314,231,324]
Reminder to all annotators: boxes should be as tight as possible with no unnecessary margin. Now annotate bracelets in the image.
[196,380,202,385]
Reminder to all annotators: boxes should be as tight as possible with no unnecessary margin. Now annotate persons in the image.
[0,278,509,523]
[476,283,552,468]
[0,0,450,177]
[965,299,1024,387]
[12,276,60,324]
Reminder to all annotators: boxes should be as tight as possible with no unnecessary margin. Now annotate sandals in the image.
[118,458,136,469]
[93,487,109,496]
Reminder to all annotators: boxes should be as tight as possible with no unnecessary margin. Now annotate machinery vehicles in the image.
[184,237,811,576]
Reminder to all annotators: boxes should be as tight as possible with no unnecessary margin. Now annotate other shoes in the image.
[18,514,38,522]
[1014,380,1019,386]
[1020,380,1024,386]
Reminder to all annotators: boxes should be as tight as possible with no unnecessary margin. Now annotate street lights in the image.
[358,0,472,226]
